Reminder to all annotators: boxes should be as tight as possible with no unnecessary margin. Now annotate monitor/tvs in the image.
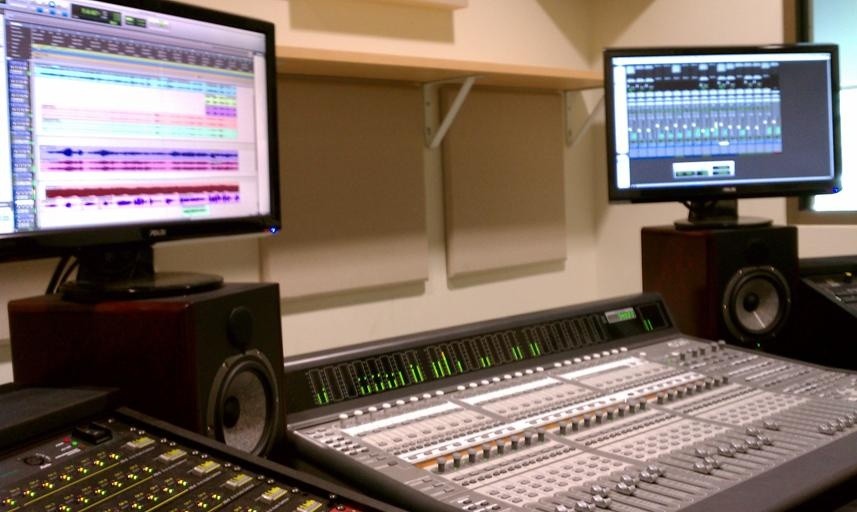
[0,0,285,305]
[603,43,841,232]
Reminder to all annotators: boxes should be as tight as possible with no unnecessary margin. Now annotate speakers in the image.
[8,281,289,469]
[639,225,803,360]
